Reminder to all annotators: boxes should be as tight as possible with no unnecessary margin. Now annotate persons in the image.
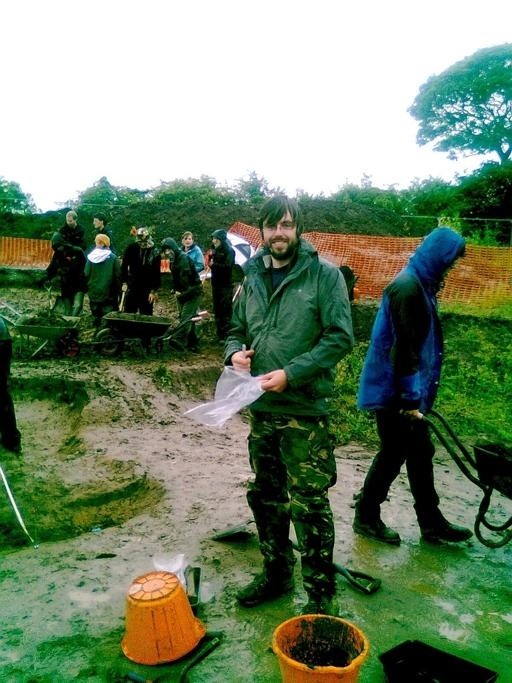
[178,232,205,274]
[83,233,122,324]
[0,316,23,453]
[225,195,354,623]
[91,213,117,253]
[354,228,476,546]
[120,227,161,348]
[161,237,200,351]
[56,210,87,249]
[208,230,238,340]
[47,232,86,318]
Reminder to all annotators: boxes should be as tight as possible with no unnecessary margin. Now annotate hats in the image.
[96,234,111,247]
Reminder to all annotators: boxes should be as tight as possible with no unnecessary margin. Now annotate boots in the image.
[351,503,402,545]
[413,503,474,545]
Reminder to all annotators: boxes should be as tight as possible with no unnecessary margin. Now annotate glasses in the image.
[263,222,297,230]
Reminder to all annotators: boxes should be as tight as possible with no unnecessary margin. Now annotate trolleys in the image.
[95,312,210,356]
[1,301,82,359]
[399,407,512,548]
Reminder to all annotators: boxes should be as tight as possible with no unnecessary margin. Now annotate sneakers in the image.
[303,592,339,618]
[139,337,225,353]
[237,570,296,608]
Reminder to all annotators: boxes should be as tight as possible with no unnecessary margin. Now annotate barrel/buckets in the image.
[122,571,207,665]
[271,614,369,682]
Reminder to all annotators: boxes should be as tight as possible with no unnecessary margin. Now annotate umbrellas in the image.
[222,228,256,270]
